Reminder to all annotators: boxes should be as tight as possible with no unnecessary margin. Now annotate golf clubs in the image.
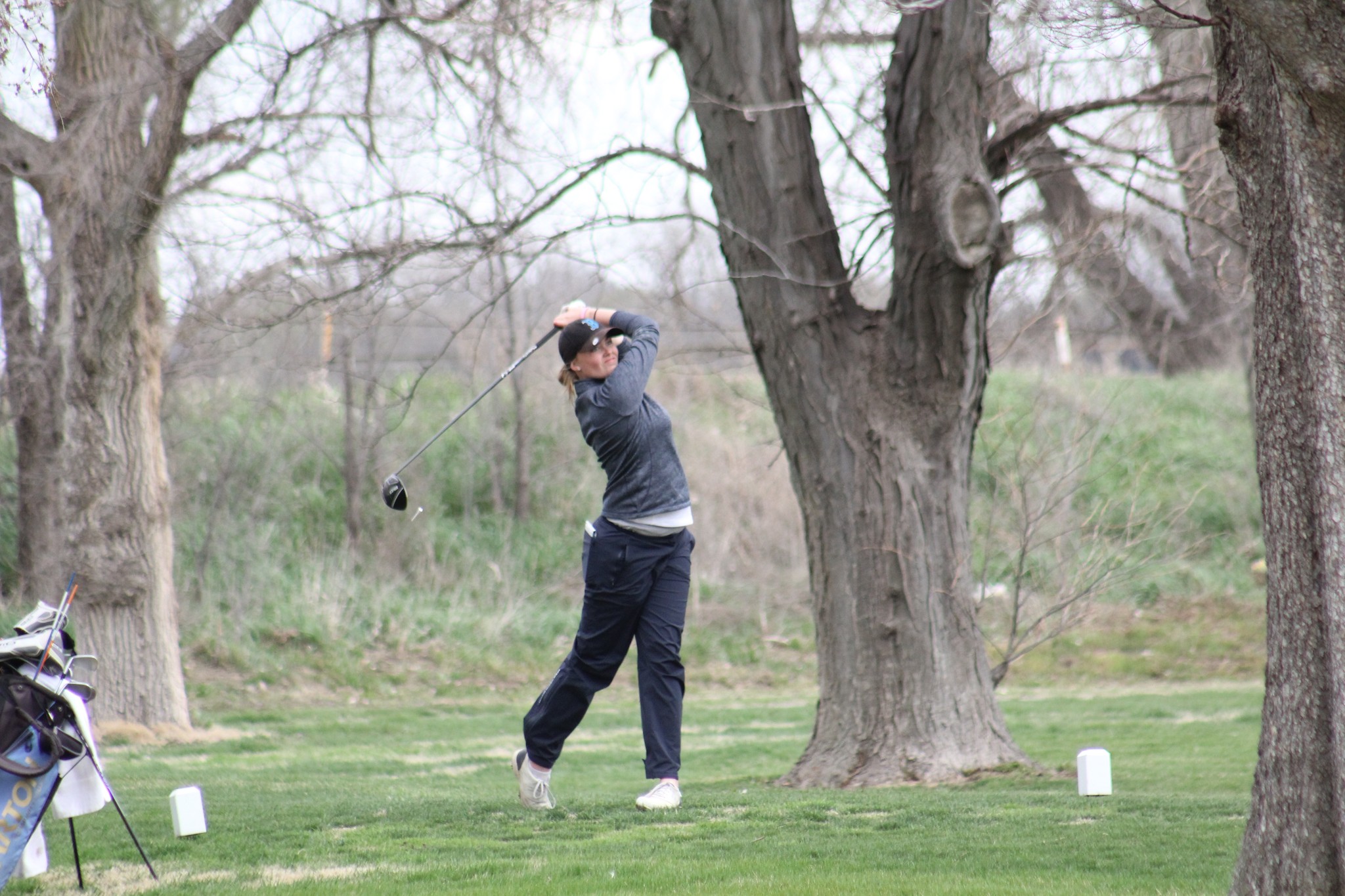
[380,324,563,511]
[50,651,159,891]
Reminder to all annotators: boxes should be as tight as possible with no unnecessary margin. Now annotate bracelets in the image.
[594,309,598,320]
[581,307,590,319]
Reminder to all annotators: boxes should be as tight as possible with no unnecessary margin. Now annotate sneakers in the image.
[512,749,556,811]
[635,782,682,810]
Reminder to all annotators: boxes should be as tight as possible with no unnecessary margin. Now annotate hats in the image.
[558,319,624,366]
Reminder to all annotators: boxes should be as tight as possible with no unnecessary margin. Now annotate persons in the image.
[515,305,696,813]
